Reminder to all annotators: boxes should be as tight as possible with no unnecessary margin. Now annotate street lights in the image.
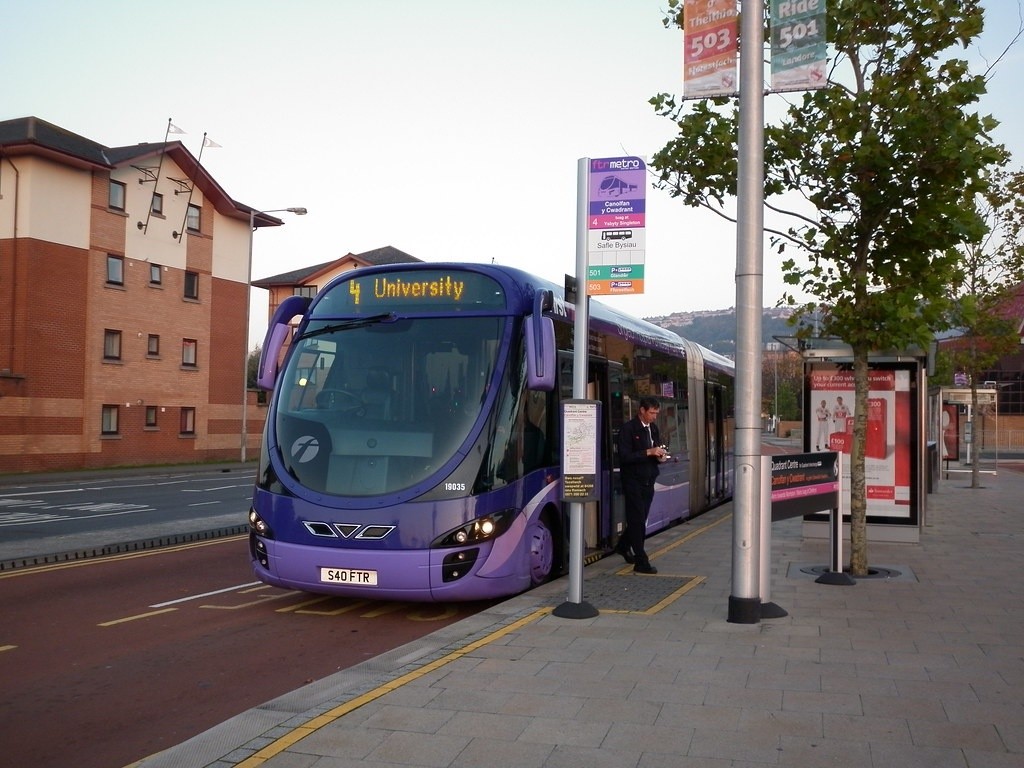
[239,206,310,462]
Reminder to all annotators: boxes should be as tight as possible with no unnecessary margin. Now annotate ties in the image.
[645,427,652,449]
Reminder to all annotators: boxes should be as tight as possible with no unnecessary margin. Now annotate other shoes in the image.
[825,445,830,448]
[615,544,636,563]
[816,446,820,451]
[634,564,657,574]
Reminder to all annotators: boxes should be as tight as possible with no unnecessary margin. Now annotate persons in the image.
[833,397,851,432]
[624,394,636,419]
[615,398,667,574]
[816,400,831,451]
[496,401,544,483]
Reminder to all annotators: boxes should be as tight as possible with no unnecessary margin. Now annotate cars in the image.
[984,380,997,389]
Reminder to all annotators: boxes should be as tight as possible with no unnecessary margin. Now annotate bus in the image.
[246,264,737,603]
